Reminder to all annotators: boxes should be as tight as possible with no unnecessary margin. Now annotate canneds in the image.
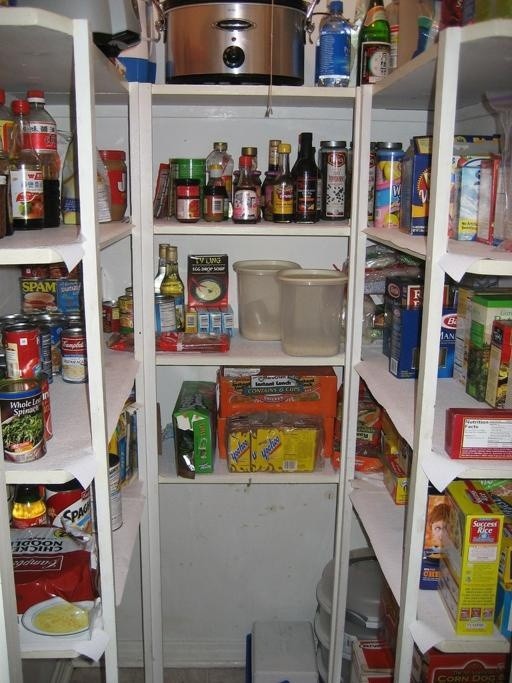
[168,158,284,225]
[97,149,128,222]
[318,142,403,228]
[0,286,178,463]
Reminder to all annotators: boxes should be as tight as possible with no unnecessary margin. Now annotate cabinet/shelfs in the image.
[1,0,150,681]
[330,12,511,682]
[139,81,361,683]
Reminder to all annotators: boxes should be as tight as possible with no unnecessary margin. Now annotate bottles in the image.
[205,129,353,223]
[159,245,186,334]
[0,86,60,239]
[312,0,399,89]
[12,484,48,530]
[368,137,404,234]
[153,242,170,296]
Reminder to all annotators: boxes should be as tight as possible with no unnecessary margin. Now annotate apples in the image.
[17,202,31,215]
[28,202,43,218]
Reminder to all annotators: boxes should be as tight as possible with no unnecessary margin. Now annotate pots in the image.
[157,0,310,85]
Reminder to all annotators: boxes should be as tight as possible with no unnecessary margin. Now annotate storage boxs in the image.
[311,544,389,682]
[215,403,338,458]
[385,0,435,75]
[386,276,512,461]
[417,478,512,635]
[351,638,394,682]
[216,365,338,418]
[403,134,502,244]
[381,587,512,680]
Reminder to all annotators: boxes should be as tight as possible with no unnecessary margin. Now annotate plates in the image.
[22,595,92,637]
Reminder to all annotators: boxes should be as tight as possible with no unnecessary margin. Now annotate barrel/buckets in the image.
[277,269,349,356]
[233,260,302,341]
